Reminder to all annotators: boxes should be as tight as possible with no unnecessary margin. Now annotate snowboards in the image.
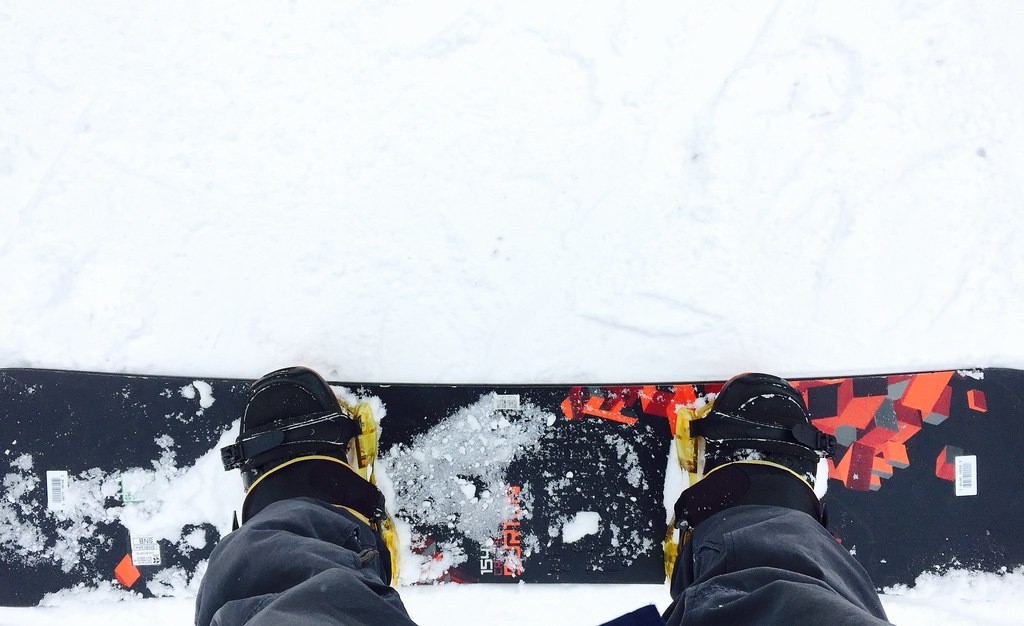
[1,366,1024,611]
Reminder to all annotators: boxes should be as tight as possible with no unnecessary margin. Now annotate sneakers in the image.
[239,366,363,496]
[697,373,818,492]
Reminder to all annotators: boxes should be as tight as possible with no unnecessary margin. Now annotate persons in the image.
[194,366,893,626]
